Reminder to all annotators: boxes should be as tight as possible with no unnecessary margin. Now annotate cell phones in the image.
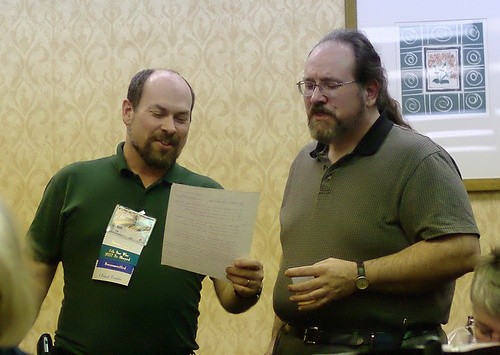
[44,337,49,353]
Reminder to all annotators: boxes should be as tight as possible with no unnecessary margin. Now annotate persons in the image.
[447,246,500,346]
[14,68,265,355]
[0,204,47,355]
[264,28,482,355]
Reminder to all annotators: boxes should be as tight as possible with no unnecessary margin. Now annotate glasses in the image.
[465,316,494,339]
[296,79,358,98]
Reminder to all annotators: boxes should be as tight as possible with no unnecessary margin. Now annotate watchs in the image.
[356,260,369,290]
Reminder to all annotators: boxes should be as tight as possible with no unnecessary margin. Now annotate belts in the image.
[282,322,441,347]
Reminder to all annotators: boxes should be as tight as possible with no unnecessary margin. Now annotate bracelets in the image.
[254,288,262,298]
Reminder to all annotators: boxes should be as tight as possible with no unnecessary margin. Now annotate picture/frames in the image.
[344,0,500,192]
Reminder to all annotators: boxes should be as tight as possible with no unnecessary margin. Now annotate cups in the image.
[291,268,316,305]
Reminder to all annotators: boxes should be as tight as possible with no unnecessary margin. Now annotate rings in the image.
[244,280,250,287]
